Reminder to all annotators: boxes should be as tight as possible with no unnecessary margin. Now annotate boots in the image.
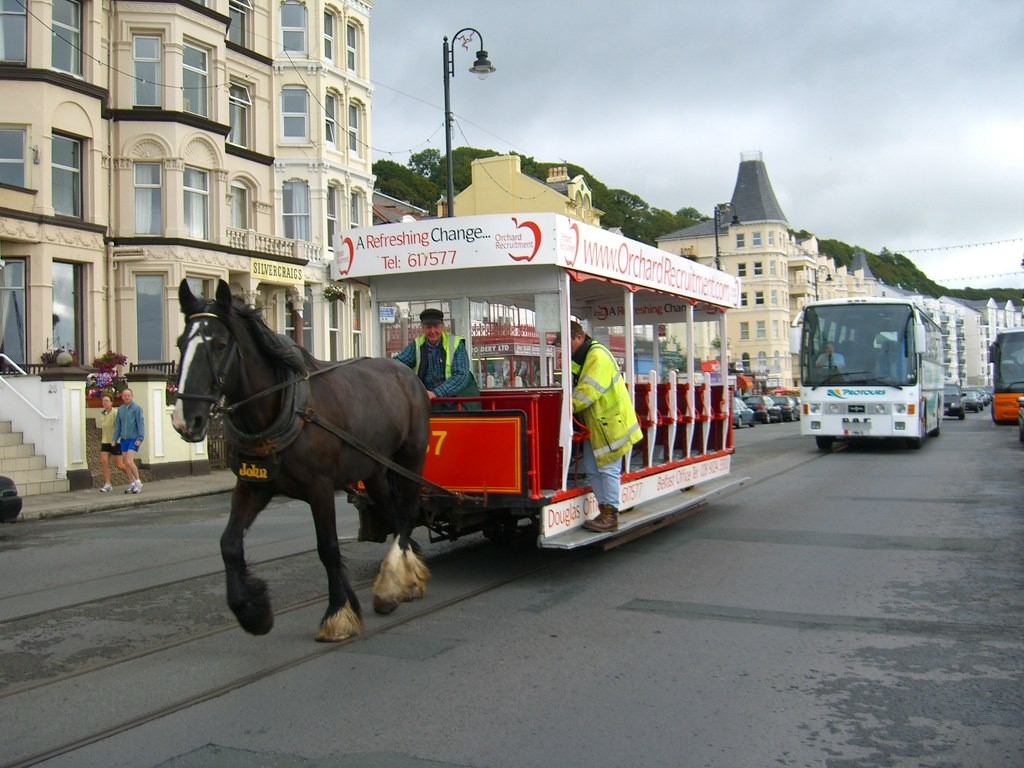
[582,504,619,532]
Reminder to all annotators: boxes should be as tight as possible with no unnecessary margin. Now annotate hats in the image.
[420,309,444,325]
[552,321,583,345]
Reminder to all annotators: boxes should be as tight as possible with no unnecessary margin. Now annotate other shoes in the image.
[100,484,113,492]
[125,482,143,494]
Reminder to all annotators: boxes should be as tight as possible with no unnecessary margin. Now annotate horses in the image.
[171,278,430,642]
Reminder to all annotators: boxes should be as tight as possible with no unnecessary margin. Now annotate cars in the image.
[964,386,993,412]
[773,397,801,421]
[943,383,967,420]
[1016,395,1024,440]
[743,395,783,424]
[730,396,756,428]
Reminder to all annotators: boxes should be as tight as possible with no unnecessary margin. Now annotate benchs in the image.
[573,382,728,486]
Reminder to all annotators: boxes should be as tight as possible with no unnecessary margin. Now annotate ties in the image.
[829,354,832,369]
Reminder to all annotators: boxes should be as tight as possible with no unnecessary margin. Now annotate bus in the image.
[791,298,946,450]
[988,328,1024,425]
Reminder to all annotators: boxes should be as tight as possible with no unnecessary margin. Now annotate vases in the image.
[167,399,176,405]
[86,388,125,407]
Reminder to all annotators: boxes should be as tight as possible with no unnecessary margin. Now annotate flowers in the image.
[41,346,79,367]
[85,351,129,399]
[166,384,179,399]
[323,285,347,305]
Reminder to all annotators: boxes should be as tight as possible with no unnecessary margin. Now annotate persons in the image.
[111,388,145,494]
[392,309,482,413]
[815,341,846,370]
[552,319,643,533]
[95,393,128,493]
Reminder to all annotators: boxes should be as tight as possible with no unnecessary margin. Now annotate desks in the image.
[480,389,564,490]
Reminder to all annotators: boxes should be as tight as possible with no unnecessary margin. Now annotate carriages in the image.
[167,210,746,641]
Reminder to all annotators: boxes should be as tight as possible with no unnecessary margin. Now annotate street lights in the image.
[714,202,744,270]
[815,264,834,300]
[441,27,498,216]
